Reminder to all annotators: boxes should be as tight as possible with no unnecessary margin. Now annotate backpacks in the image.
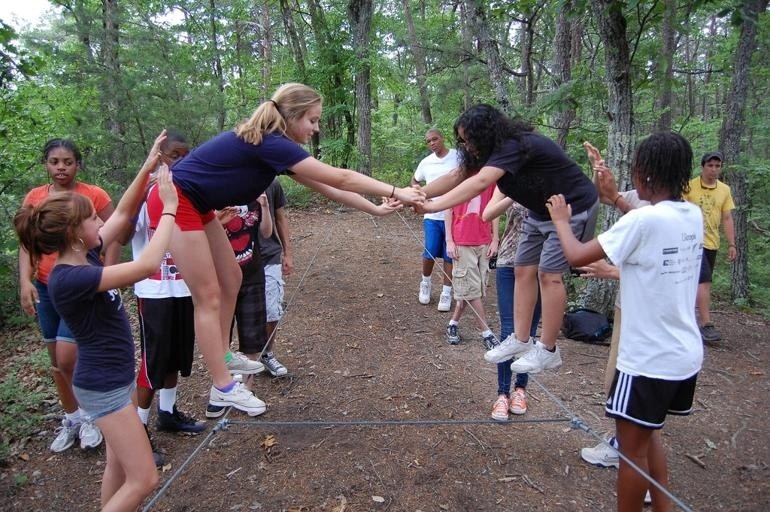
[562,308,610,346]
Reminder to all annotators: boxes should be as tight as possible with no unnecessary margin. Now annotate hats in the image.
[701,149,724,162]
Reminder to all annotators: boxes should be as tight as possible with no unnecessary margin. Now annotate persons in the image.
[583,140,653,504]
[233,178,293,382]
[12,129,180,512]
[206,191,272,418]
[148,82,424,413]
[682,153,737,341]
[546,131,704,511]
[389,104,599,373]
[18,138,121,454]
[411,129,542,424]
[118,133,207,467]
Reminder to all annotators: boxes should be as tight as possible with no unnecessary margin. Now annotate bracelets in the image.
[728,243,736,247]
[614,195,622,205]
[130,217,138,224]
[161,213,177,218]
[390,185,396,200]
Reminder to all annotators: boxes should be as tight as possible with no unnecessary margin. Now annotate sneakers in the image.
[157,404,208,433]
[224,352,265,375]
[418,280,432,304]
[491,394,509,422]
[509,387,528,415]
[699,322,722,343]
[445,323,461,345]
[437,292,452,312]
[205,374,267,418]
[581,436,620,469]
[141,423,162,466]
[78,414,104,450]
[482,333,534,364]
[261,351,288,377]
[49,415,82,454]
[509,341,563,374]
